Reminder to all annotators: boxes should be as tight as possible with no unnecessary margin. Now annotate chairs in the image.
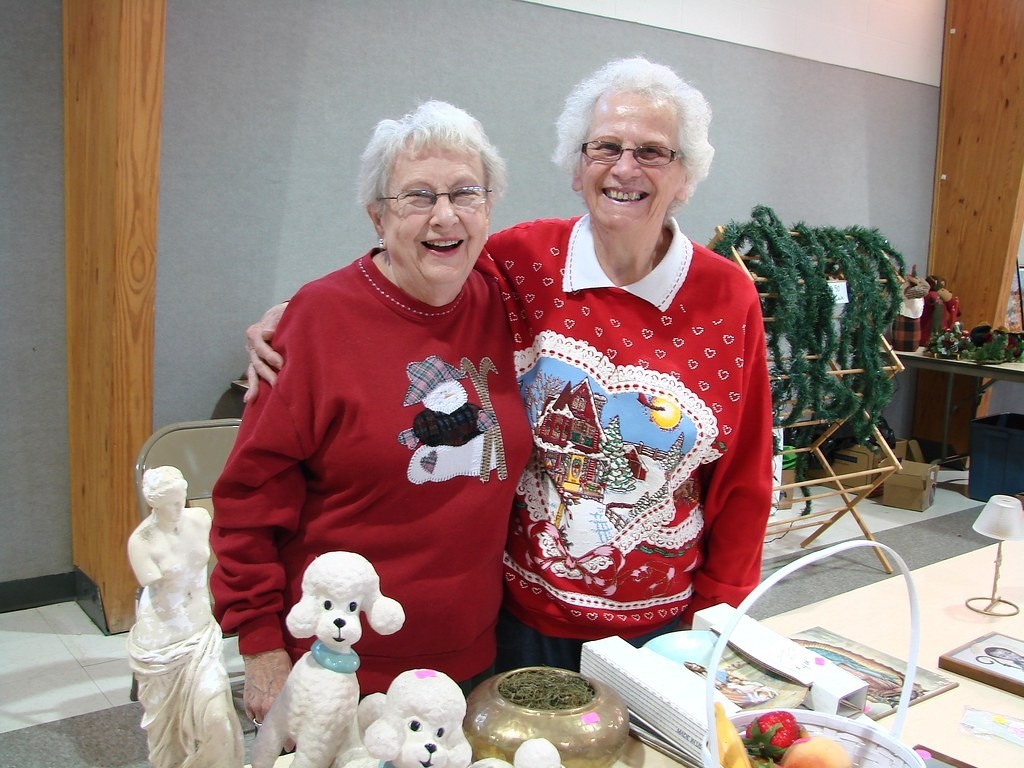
[130,417,242,703]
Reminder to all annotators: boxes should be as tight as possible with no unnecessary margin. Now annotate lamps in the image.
[965,495,1023,616]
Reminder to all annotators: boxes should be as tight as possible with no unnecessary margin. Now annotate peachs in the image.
[780,736,853,768]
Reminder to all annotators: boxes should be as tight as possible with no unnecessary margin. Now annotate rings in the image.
[253,719,262,726]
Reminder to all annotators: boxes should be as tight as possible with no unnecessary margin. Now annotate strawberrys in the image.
[740,710,801,768]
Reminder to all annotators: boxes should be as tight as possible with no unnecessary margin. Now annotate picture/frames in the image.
[939,631,1024,697]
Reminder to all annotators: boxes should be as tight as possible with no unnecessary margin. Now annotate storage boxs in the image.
[804,437,907,498]
[778,470,795,510]
[877,457,939,512]
[968,412,1024,503]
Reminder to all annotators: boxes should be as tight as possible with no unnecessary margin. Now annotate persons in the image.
[210,98,536,754]
[127,466,247,768]
[985,647,1024,672]
[244,56,774,684]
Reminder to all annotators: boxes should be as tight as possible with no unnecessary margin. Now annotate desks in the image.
[613,535,1023,768]
[880,345,1024,464]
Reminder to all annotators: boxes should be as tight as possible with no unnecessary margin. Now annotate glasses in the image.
[575,137,681,168]
[377,186,493,207]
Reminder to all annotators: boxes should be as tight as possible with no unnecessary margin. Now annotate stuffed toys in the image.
[904,273,961,316]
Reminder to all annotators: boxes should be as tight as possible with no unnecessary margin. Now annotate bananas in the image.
[713,702,751,768]
